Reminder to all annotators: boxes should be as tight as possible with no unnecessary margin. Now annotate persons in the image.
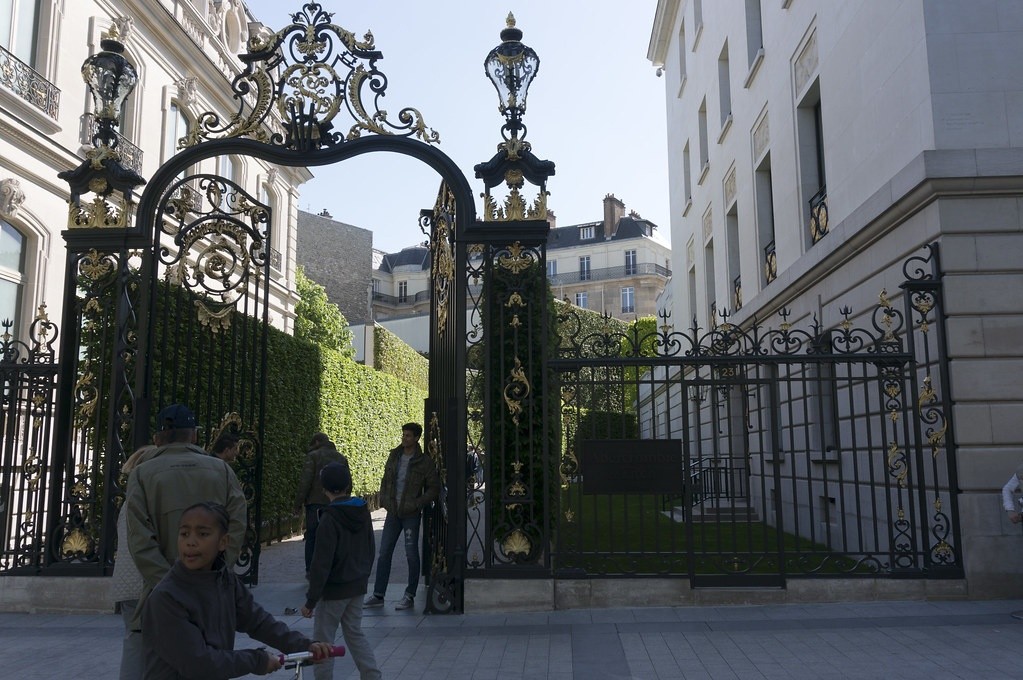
[118,502,333,680]
[294,433,350,580]
[118,406,247,627]
[1001,471,1023,619]
[303,463,382,680]
[364,422,439,610]
[466,445,484,507]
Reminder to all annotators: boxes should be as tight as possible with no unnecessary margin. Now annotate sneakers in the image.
[362,596,385,608]
[395,595,414,609]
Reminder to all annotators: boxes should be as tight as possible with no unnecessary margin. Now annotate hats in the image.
[155,404,196,432]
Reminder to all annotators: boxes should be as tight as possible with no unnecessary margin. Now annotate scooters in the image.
[272,644,346,680]
